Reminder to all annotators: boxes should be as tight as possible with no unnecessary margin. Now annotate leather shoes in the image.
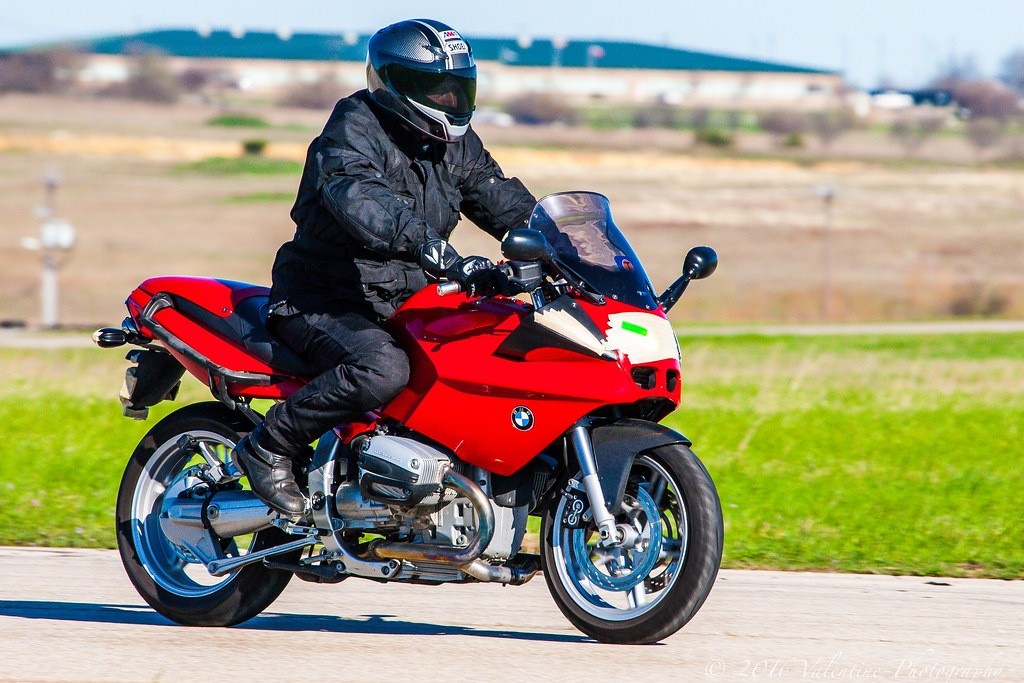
[230,422,305,516]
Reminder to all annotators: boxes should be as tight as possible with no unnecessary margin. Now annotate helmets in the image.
[365,18,476,143]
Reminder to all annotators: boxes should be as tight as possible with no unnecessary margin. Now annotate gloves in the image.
[418,239,501,298]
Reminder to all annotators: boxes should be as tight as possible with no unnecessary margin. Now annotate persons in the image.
[232,18,579,517]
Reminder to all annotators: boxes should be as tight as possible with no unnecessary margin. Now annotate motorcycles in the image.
[91,189,732,645]
